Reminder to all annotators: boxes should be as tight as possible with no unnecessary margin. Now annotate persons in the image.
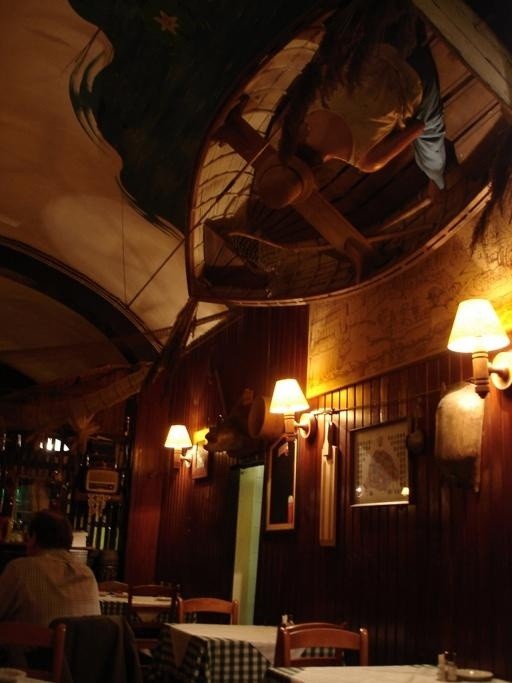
[0,511,103,673]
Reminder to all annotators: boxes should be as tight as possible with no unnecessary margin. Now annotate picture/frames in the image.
[347,416,416,508]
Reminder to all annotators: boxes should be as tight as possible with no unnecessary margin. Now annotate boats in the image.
[181,3,511,308]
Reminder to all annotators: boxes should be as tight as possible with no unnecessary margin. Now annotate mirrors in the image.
[265,438,298,532]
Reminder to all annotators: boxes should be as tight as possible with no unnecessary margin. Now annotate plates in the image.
[457,668,494,680]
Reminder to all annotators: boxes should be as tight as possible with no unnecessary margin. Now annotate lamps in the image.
[164,423,193,468]
[447,299,512,399]
[269,378,317,443]
